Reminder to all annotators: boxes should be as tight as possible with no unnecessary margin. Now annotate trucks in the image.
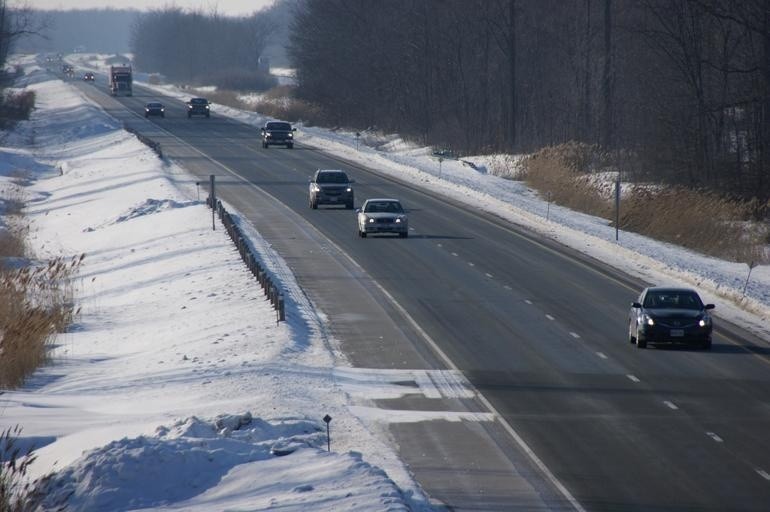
[106,64,134,97]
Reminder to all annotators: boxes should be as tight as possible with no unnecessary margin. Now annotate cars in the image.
[45,45,95,82]
[628,286,715,349]
[356,198,410,238]
[143,102,165,118]
[146,76,161,85]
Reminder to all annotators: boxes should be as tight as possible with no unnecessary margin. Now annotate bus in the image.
[257,56,269,73]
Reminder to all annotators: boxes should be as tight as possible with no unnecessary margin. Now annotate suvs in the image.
[308,169,355,209]
[261,120,296,149]
[184,98,212,119]
